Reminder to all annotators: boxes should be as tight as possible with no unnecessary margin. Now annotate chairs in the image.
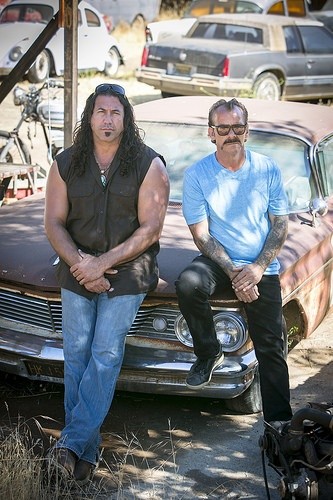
[234,32,254,41]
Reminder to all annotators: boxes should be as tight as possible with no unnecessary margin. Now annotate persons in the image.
[176,98,294,426]
[42,83,171,484]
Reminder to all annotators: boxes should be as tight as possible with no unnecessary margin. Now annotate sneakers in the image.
[185,348,225,390]
[71,460,92,487]
[47,448,76,480]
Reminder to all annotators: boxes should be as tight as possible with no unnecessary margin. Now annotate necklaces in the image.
[94,151,112,186]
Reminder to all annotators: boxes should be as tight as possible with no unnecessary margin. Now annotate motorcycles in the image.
[0,78,85,208]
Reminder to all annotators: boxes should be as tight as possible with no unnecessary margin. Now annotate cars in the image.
[134,14,333,101]
[176,0,333,37]
[1,95,333,415]
[83,1,163,31]
[0,0,125,79]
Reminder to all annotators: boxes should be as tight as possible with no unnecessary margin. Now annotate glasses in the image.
[95,84,126,96]
[211,123,247,136]
